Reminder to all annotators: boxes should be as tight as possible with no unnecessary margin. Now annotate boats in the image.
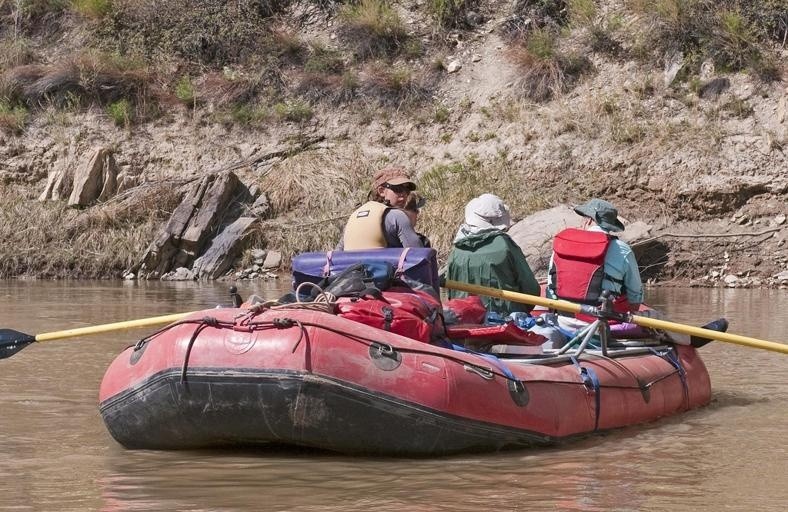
[98,278,714,459]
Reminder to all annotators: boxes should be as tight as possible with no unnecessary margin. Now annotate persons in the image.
[443,193,541,317]
[402,190,431,249]
[547,198,729,348]
[334,166,423,249]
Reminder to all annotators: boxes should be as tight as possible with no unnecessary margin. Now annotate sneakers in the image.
[693,318,728,348]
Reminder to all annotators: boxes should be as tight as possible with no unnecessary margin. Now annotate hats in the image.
[372,168,416,191]
[573,197,626,232]
[464,193,510,232]
[403,192,426,210]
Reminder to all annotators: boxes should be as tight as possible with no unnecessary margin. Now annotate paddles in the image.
[0,286,243,359]
